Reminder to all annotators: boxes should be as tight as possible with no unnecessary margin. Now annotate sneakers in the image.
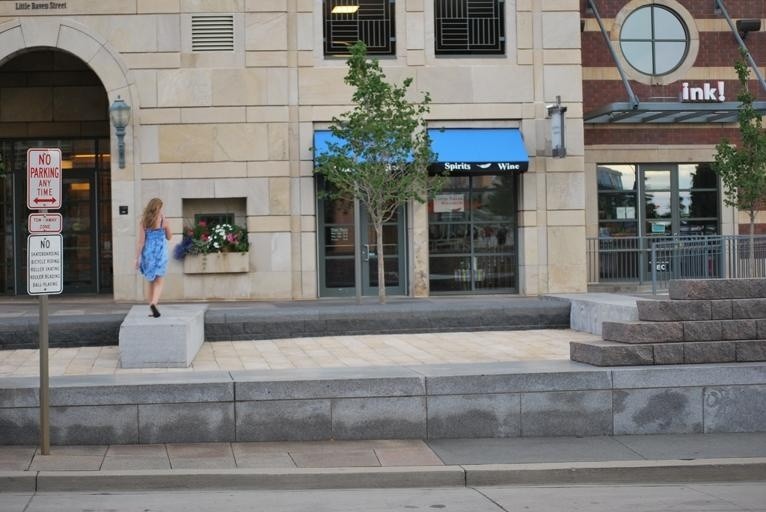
[148,305,160,318]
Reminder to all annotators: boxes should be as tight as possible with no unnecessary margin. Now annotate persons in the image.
[135,197,172,318]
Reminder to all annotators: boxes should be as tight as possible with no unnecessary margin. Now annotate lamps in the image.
[546,94,568,159]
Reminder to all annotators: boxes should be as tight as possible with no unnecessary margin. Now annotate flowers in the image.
[174,208,248,260]
